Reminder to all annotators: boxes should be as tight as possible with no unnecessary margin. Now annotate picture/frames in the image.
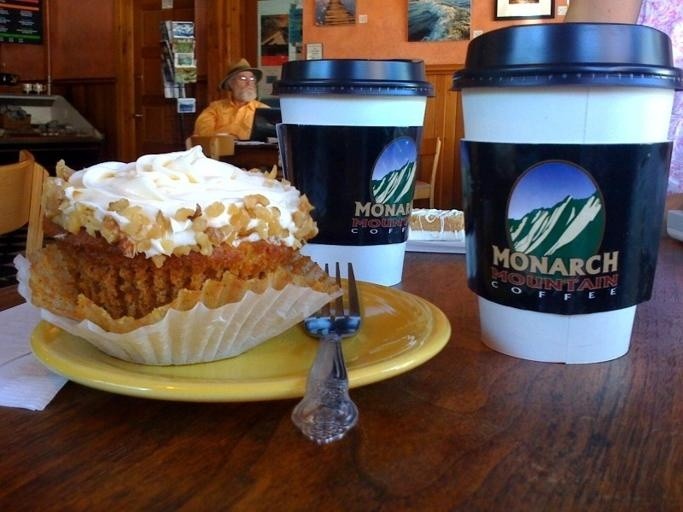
[493,0,557,22]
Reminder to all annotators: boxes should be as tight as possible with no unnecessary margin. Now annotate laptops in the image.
[237,107,281,142]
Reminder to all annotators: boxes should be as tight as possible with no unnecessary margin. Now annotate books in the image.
[158,19,199,85]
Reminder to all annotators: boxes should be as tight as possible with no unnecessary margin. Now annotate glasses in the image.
[230,75,257,82]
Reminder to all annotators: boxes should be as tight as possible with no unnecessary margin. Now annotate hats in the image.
[218,57,263,92]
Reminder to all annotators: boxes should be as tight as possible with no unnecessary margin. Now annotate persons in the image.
[186,55,273,143]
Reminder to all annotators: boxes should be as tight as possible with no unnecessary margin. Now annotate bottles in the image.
[23,83,44,95]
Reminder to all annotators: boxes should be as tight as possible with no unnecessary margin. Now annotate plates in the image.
[31,275,452,404]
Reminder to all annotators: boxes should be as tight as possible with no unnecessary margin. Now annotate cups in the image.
[450,26,680,365]
[282,58,435,287]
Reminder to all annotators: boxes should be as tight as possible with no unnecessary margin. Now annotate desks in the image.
[221,139,281,171]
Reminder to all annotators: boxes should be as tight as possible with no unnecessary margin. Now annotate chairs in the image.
[1,148,51,282]
[412,136,445,209]
[184,134,235,161]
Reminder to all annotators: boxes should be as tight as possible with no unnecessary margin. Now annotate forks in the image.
[290,263,358,444]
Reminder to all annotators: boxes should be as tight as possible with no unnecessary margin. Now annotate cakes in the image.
[408,208,464,241]
[42,145,319,320]
